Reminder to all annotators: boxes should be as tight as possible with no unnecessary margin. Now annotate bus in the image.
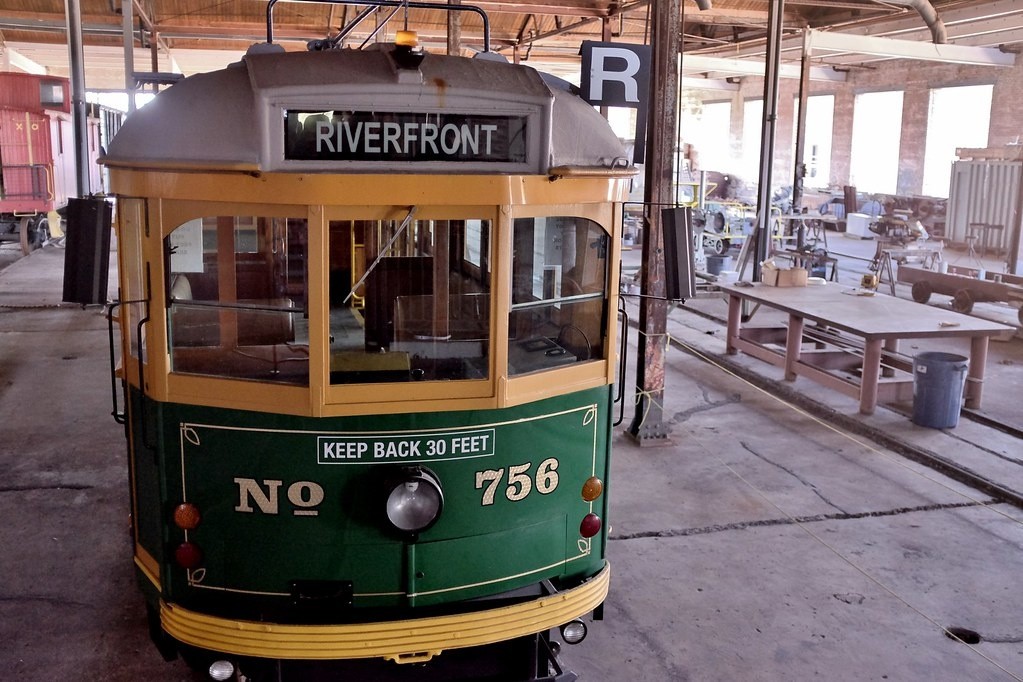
[60,0,696,682]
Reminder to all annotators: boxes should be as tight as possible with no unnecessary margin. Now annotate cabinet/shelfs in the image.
[846,213,883,239]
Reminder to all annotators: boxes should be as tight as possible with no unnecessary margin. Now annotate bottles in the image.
[625,234,633,246]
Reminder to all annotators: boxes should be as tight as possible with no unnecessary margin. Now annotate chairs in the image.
[172,299,295,345]
[393,294,483,345]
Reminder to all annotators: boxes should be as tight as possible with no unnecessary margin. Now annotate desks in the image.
[721,281,1016,415]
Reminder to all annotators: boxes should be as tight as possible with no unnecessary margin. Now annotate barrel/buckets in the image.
[707,255,733,275]
[912,352,970,428]
[937,260,948,274]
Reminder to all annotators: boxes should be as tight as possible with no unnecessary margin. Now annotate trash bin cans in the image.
[910,352,970,429]
[706,254,733,275]
